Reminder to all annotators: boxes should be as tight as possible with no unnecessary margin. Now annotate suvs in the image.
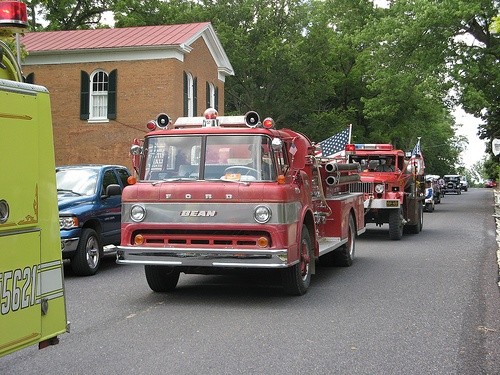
[55,163,133,277]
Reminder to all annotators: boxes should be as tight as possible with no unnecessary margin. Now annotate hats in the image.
[249,145,263,153]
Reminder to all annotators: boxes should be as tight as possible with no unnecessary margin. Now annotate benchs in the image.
[178,165,238,179]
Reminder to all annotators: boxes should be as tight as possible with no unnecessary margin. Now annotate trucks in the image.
[0,0,72,359]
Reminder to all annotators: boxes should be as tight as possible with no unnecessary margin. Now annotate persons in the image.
[376,157,402,172]
[238,144,274,180]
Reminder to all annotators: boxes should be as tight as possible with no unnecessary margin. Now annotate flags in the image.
[314,128,349,164]
[407,142,426,173]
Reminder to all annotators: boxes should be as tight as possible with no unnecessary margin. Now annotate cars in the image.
[423,174,469,213]
[483,179,497,188]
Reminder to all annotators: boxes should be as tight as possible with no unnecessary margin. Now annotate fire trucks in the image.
[115,107,368,297]
[341,143,427,242]
